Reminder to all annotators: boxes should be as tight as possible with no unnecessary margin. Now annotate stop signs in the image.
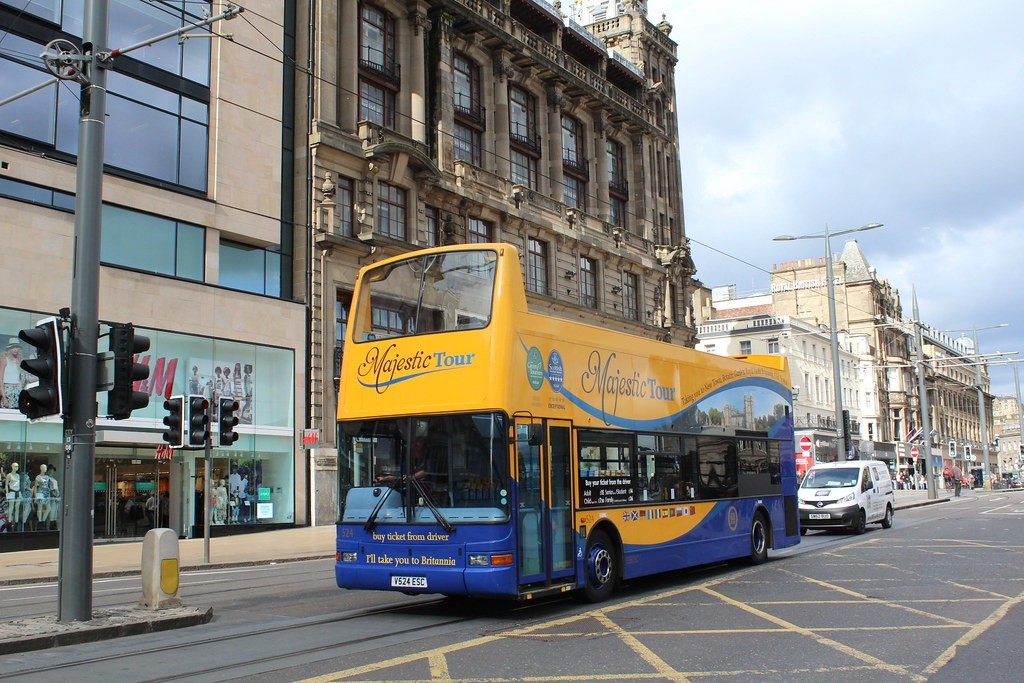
[800,436,812,451]
[911,447,919,457]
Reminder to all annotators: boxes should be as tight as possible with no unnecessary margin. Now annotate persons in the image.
[215,479,228,524]
[145,491,159,530]
[21,464,33,532]
[892,463,978,497]
[210,479,216,526]
[374,438,432,486]
[229,462,251,524]
[5,462,21,531]
[0,337,27,409]
[190,362,253,421]
[47,465,60,530]
[34,464,51,531]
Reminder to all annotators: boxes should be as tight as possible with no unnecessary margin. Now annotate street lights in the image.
[772,222,883,460]
[942,323,1010,490]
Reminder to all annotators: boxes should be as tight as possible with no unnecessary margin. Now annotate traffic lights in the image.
[1020,444,1024,461]
[188,394,209,447]
[964,446,972,461]
[949,441,956,457]
[163,395,184,448]
[108,323,150,419]
[18,316,63,421]
[219,397,239,446]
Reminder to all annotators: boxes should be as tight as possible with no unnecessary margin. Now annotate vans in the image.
[797,461,894,535]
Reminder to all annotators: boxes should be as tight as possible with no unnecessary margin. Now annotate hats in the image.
[47,464,56,471]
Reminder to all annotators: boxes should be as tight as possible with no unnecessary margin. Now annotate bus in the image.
[335,243,801,608]
